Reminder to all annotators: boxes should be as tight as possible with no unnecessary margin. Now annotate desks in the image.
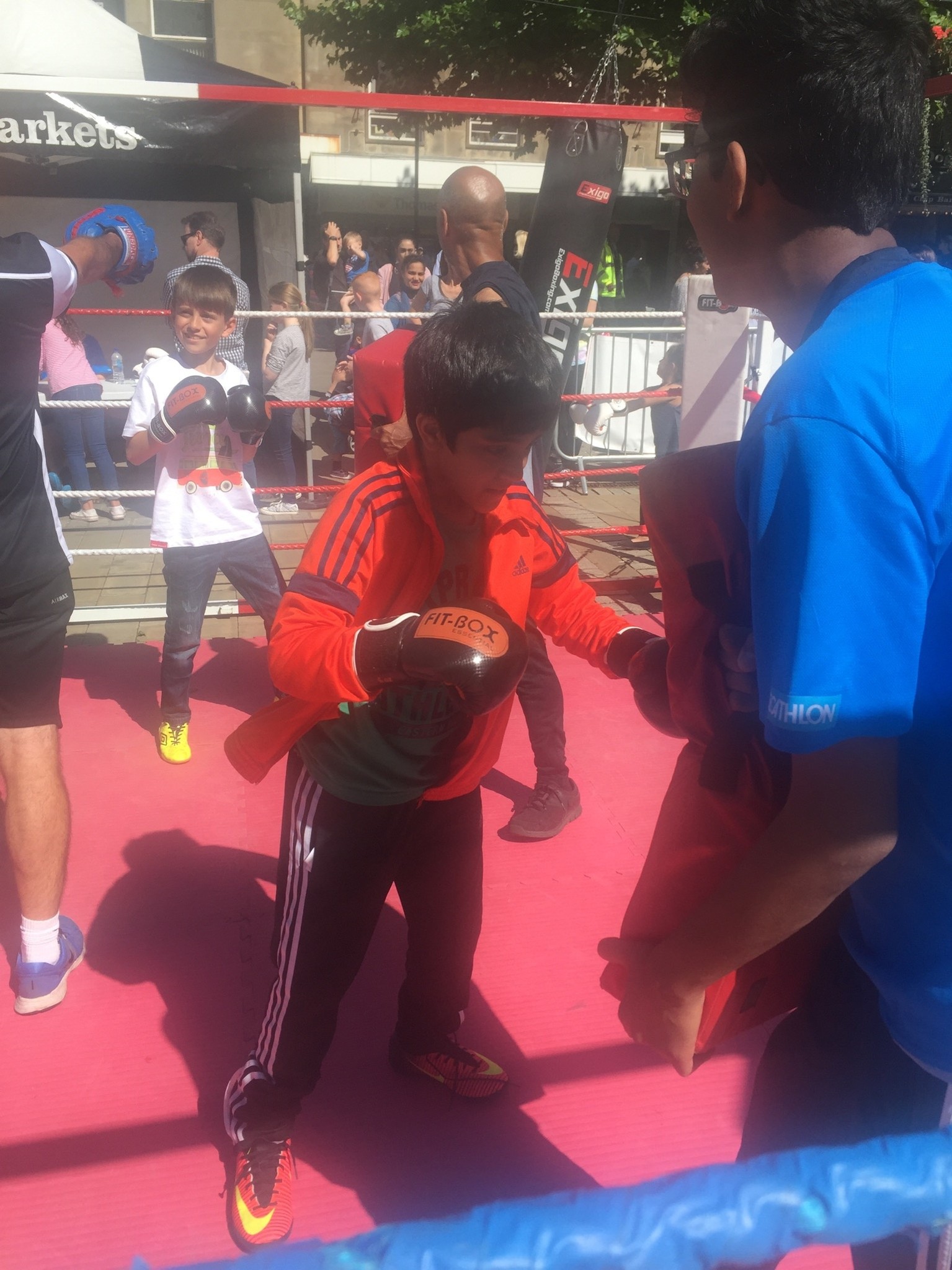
[38,380,137,400]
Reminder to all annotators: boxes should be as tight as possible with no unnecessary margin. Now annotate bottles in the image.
[111,348,124,384]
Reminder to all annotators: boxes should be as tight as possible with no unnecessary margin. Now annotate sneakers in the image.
[508,778,583,839]
[158,721,192,765]
[259,500,299,516]
[389,1039,511,1105]
[15,913,87,1015]
[550,468,577,487]
[275,491,301,499]
[228,1133,298,1255]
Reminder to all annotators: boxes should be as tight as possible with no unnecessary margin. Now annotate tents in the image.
[0,0,315,502]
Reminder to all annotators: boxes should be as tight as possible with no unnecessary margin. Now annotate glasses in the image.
[663,137,736,199]
[179,231,196,244]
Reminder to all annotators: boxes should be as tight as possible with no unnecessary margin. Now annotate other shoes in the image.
[333,324,354,336]
[108,505,126,520]
[330,468,355,480]
[69,509,98,522]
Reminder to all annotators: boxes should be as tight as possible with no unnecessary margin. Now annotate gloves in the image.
[569,399,612,425]
[133,348,170,378]
[607,630,687,740]
[228,385,273,446]
[62,205,157,284]
[583,399,629,436]
[354,596,528,718]
[148,375,228,445]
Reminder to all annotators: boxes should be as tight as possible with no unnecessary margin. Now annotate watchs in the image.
[329,236,338,240]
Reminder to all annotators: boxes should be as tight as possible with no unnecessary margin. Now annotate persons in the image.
[39,313,127,521]
[303,222,465,480]
[259,281,316,517]
[121,266,290,764]
[164,211,258,504]
[543,282,597,488]
[0,203,157,1016]
[599,239,623,334]
[660,249,711,344]
[513,229,528,258]
[569,343,683,460]
[598,0,952,1270]
[222,300,687,1255]
